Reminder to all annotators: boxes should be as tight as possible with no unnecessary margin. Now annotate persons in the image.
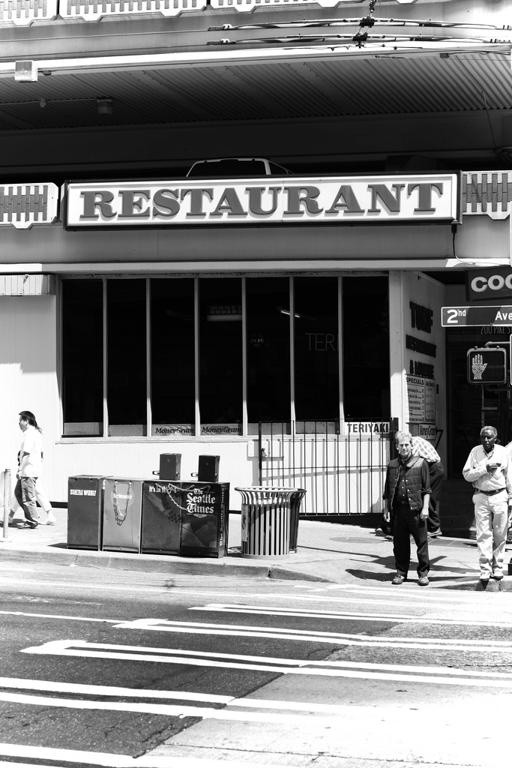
[501,440,511,578]
[461,423,512,591]
[400,429,444,539]
[16,430,56,528]
[379,427,433,586]
[15,407,43,533]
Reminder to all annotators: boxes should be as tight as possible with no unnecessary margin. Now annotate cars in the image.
[185,158,294,179]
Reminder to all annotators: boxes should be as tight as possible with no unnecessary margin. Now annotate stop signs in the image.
[466,347,507,385]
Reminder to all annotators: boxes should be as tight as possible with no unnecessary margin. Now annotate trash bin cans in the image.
[234,486,307,559]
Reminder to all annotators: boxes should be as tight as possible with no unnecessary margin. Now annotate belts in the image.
[473,487,507,497]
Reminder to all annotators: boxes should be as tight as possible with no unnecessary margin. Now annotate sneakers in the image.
[417,574,430,586]
[391,573,407,584]
[479,566,505,581]
